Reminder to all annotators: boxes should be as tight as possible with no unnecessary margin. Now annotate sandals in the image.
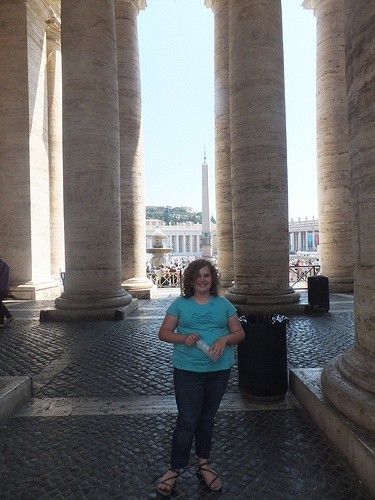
[157,468,180,496]
[198,462,222,492]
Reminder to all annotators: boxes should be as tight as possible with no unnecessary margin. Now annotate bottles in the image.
[194,338,219,361]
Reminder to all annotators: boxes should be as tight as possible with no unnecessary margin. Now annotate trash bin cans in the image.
[308,276,330,312]
[239,313,288,404]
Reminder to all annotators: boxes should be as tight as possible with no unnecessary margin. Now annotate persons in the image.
[0,259,14,326]
[157,259,246,496]
[143,252,320,289]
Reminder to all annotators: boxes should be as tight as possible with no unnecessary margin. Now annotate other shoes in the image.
[0,324,5,327]
[7,317,14,324]
